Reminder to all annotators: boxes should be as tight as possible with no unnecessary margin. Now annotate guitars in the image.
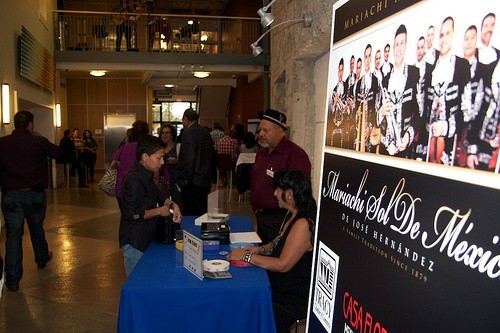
[112,6,145,27]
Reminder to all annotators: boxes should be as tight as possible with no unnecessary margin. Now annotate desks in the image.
[117,213,278,333]
[72,147,90,186]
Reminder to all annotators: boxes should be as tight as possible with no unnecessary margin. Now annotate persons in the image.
[175,17,199,39]
[148,16,156,52]
[110,120,171,248]
[204,122,255,187]
[325,13,500,173]
[226,169,317,333]
[250,109,311,241]
[60,128,98,188]
[0,110,77,291]
[173,108,217,216]
[158,122,181,244]
[119,135,182,278]
[112,2,131,51]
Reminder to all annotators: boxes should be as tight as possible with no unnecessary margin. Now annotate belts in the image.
[255,207,289,216]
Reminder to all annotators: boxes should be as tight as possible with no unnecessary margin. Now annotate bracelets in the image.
[243,251,252,263]
[170,203,173,209]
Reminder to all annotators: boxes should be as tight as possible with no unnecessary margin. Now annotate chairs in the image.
[215,154,257,204]
[60,149,79,189]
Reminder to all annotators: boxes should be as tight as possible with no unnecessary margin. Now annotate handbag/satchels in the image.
[156,202,182,245]
[98,169,117,198]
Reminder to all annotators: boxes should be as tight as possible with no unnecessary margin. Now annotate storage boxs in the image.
[201,223,230,244]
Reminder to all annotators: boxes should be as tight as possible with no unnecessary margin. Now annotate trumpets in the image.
[332,91,350,115]
[379,82,401,148]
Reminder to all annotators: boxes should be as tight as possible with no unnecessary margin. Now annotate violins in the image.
[430,108,444,163]
[455,126,468,167]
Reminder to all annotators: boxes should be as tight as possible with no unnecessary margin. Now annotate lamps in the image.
[55,103,62,128]
[2,83,11,125]
[256,0,279,27]
[250,14,313,58]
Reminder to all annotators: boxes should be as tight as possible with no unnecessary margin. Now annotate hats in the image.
[260,109,288,131]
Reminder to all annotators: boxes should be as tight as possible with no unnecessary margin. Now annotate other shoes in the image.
[37,252,53,270]
[6,284,19,291]
